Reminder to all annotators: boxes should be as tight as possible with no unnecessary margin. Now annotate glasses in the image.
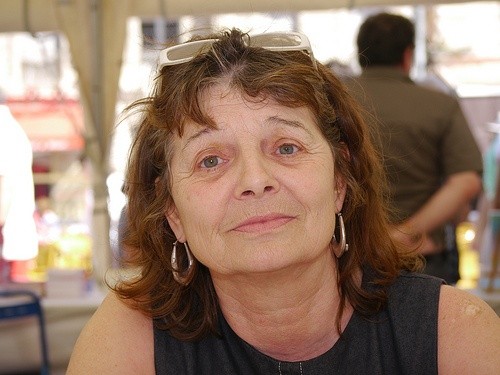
[157,31,320,96]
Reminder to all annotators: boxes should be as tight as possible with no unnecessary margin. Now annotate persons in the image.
[64,27,498,375]
[333,11,486,287]
